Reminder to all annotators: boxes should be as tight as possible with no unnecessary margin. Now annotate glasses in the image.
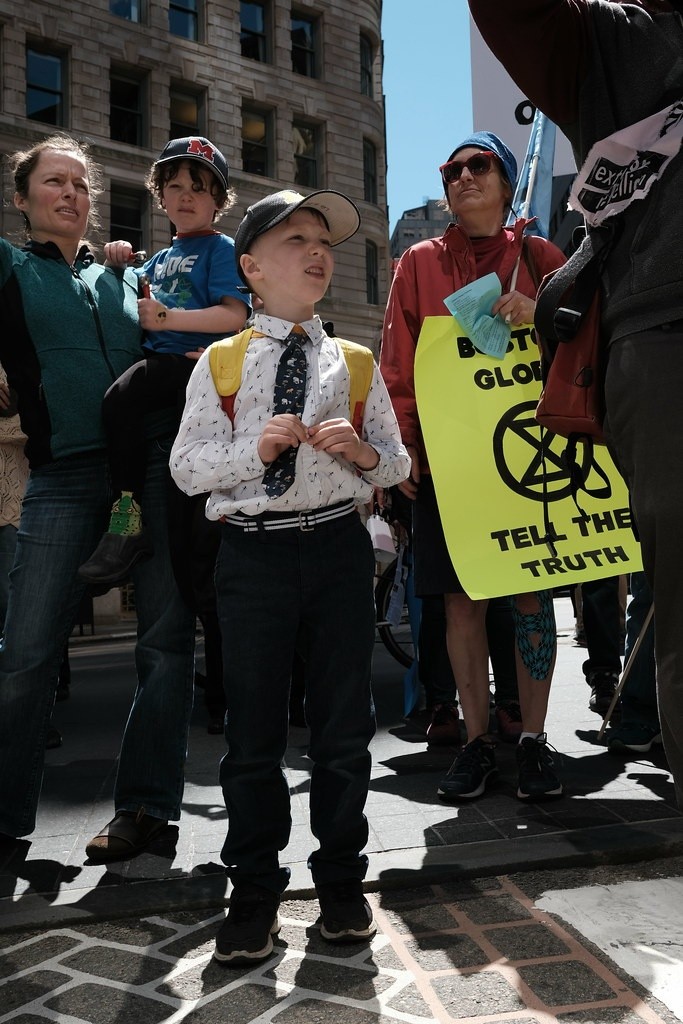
[440,150,501,184]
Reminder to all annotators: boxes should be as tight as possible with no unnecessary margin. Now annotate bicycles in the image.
[377,536,420,679]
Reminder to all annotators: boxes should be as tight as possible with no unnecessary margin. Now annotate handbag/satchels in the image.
[535,282,605,445]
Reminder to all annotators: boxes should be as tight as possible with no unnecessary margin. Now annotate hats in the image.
[156,136,229,193]
[442,131,517,208]
[235,189,361,294]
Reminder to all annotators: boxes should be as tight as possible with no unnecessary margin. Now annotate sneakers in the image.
[78,534,150,584]
[607,722,663,751]
[315,878,376,939]
[516,732,563,798]
[423,700,462,745]
[593,683,621,711]
[495,699,523,743]
[437,733,500,800]
[214,869,290,964]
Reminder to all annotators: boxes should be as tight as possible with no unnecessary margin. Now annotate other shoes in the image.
[85,806,175,858]
[207,718,224,733]
[55,685,70,701]
[0,838,32,861]
[45,724,63,749]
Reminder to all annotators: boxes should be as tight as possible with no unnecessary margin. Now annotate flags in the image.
[506,110,556,239]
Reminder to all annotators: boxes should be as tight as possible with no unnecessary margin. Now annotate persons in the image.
[468,0,683,810]
[76,136,253,584]
[0,131,197,870]
[377,131,569,800]
[168,189,412,966]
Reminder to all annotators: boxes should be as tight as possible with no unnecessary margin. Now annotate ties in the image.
[262,333,311,500]
